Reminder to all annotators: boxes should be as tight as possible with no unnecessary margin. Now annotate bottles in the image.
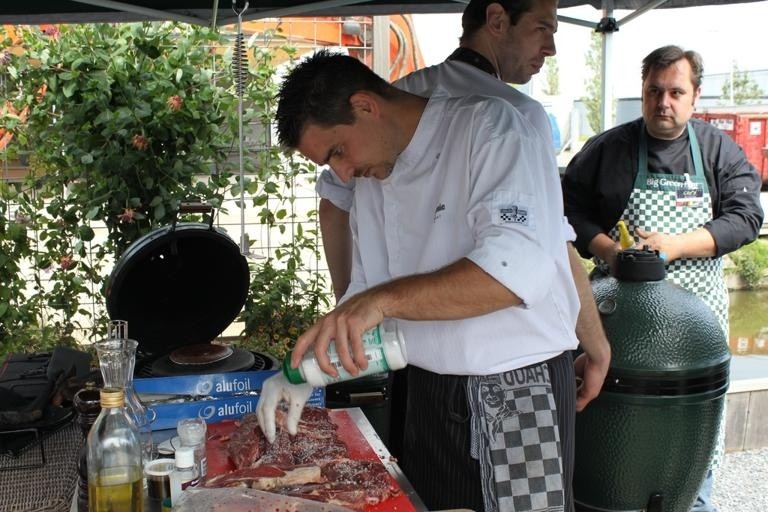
[282,318,410,388]
[71,317,144,512]
[616,218,637,253]
[149,417,208,512]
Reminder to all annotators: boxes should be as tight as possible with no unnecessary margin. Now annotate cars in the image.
[554,134,594,172]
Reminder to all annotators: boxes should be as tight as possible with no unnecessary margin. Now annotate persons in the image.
[254,44,581,511]
[560,46,763,510]
[312,0,612,459]
